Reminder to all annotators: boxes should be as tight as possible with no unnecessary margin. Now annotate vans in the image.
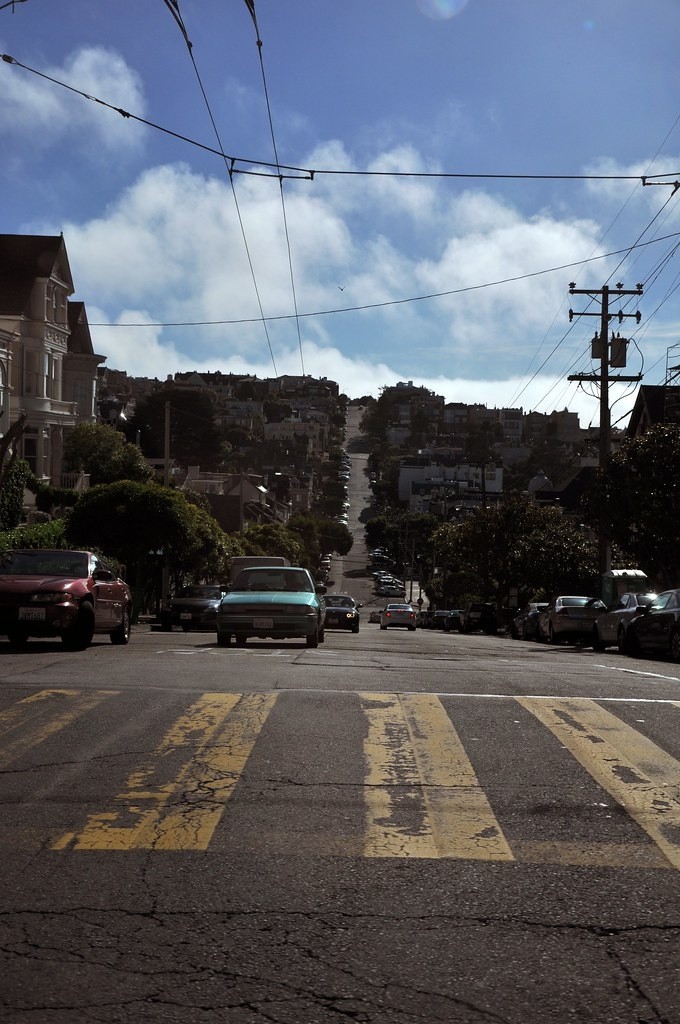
[230,554,294,590]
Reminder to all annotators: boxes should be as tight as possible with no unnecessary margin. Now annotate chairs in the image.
[284,574,306,592]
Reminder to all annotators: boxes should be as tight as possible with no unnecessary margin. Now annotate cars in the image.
[417,600,496,634]
[161,585,226,632]
[509,601,551,639]
[379,601,418,633]
[369,543,408,597]
[619,588,680,663]
[321,594,363,633]
[370,610,383,622]
[536,595,609,645]
[0,548,134,653]
[314,401,352,595]
[216,565,328,649]
[589,590,657,651]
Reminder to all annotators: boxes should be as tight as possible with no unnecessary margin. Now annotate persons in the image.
[284,573,298,590]
